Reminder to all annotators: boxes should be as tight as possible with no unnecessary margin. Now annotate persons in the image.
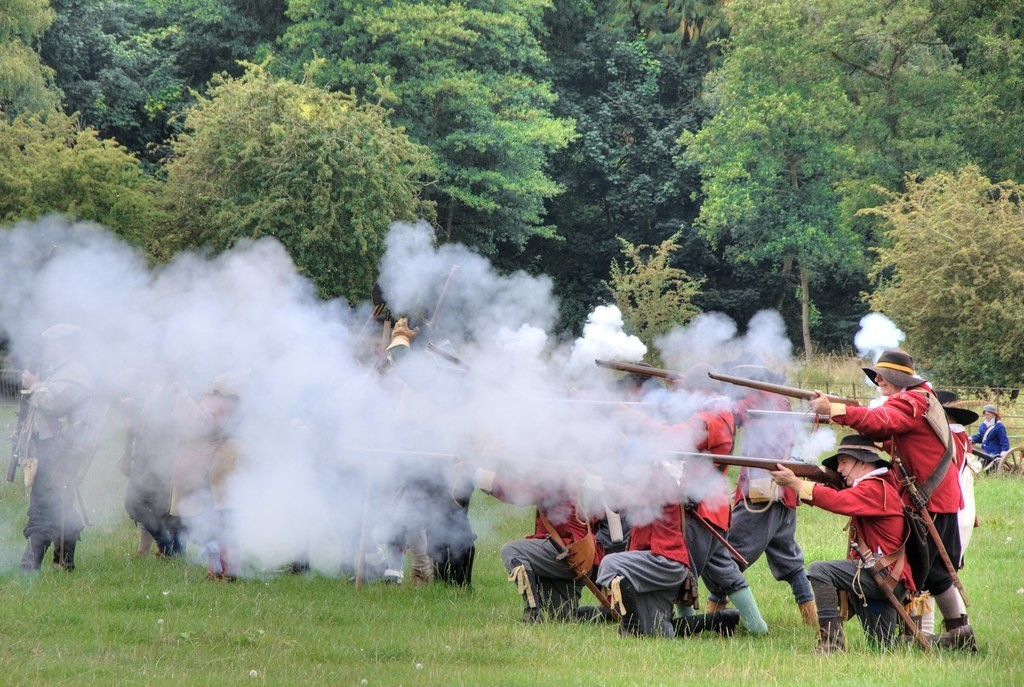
[771,432,982,652]
[969,404,1012,468]
[10,301,770,639]
[807,347,975,654]
[919,386,980,636]
[662,346,823,629]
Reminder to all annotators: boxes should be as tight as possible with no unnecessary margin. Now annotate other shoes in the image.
[384,570,404,585]
[412,569,429,586]
[366,548,386,579]
[522,607,544,624]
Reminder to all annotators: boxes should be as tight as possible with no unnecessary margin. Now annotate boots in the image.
[433,542,475,592]
[609,578,646,638]
[907,625,979,653]
[942,614,967,632]
[706,600,726,613]
[799,600,818,626]
[815,617,847,652]
[671,609,740,638]
[20,532,52,571]
[899,615,922,636]
[54,537,77,572]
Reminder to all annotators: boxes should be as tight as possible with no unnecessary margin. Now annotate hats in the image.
[822,435,895,470]
[606,363,664,393]
[203,372,241,397]
[936,391,979,426]
[983,405,996,413]
[862,349,927,387]
[682,363,721,393]
[723,352,787,386]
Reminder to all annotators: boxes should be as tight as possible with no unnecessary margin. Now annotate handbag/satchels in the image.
[749,478,778,504]
[885,480,929,590]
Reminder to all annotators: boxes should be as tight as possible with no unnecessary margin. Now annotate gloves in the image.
[385,317,422,350]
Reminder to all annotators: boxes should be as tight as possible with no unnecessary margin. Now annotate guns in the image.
[666,450,843,488]
[595,358,683,381]
[422,264,457,332]
[348,302,392,369]
[427,341,472,373]
[347,446,464,464]
[116,401,136,476]
[4,347,39,483]
[707,371,860,407]
[744,406,831,425]
